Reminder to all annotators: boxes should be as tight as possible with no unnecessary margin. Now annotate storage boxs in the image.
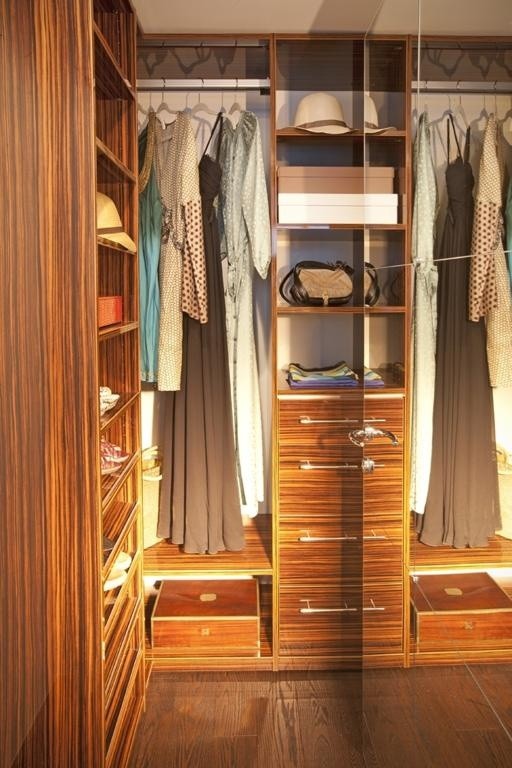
[150,581,260,654]
[411,572,511,648]
[277,164,396,194]
[278,189,398,226]
[97,295,125,329]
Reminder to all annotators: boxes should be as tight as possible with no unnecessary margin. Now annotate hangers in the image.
[412,77,512,131]
[136,75,260,140]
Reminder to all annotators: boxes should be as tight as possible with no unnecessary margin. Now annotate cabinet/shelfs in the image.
[409,531,512,667]
[2,0,156,767]
[271,33,410,671]
[145,525,271,675]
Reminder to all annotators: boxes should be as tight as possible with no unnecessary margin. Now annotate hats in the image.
[364,92,397,133]
[281,92,360,135]
[95,188,137,253]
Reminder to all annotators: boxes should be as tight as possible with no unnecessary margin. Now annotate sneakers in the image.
[98,434,128,475]
[98,381,121,416]
[104,552,132,592]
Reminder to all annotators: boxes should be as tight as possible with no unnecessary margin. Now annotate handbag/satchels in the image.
[290,262,355,306]
[143,446,164,551]
[494,442,512,546]
[364,262,382,306]
